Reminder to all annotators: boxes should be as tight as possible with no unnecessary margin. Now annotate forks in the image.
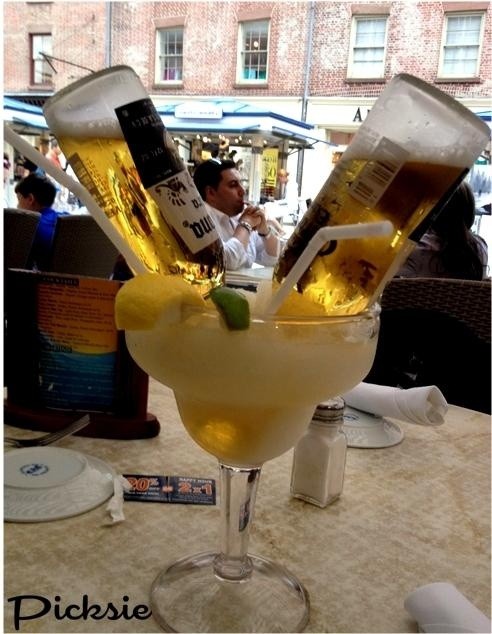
[5,417,94,445]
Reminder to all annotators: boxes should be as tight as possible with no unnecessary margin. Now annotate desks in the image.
[227,266,275,290]
[4,379,492,633]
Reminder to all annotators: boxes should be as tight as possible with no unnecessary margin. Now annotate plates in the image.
[5,447,120,522]
[338,404,404,450]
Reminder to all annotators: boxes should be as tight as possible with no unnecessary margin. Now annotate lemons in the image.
[115,270,208,331]
[271,282,346,342]
[208,286,250,331]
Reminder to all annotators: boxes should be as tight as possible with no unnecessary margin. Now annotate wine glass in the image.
[126,276,383,632]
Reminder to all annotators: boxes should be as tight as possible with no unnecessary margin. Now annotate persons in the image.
[396,181,488,281]
[277,169,301,222]
[4,138,93,214]
[193,158,281,271]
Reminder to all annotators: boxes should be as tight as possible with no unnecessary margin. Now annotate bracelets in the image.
[238,221,253,235]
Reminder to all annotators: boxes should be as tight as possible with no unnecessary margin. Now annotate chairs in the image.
[4,207,133,280]
[370,278,490,415]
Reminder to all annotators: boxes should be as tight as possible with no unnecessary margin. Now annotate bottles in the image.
[43,64,492,316]
[289,394,348,510]
